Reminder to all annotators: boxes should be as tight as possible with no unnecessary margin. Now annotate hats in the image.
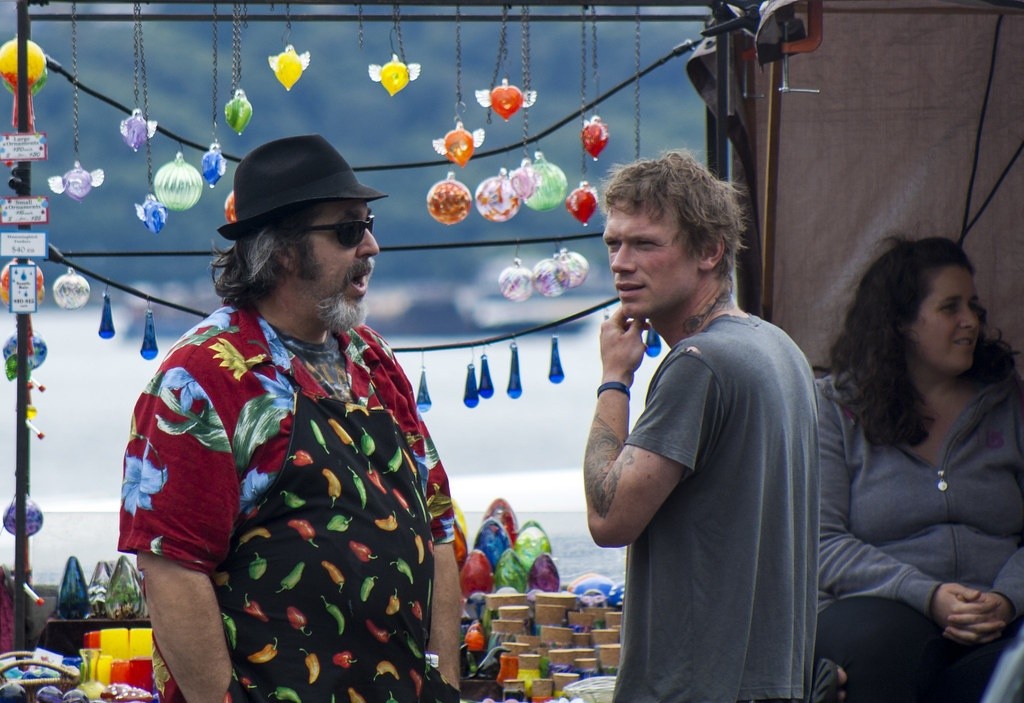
[216,134,390,241]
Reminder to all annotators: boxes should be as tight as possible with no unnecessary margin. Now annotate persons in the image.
[118,134,460,703]
[583,150,820,703]
[814,237,1024,702]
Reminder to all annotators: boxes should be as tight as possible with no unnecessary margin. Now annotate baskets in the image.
[0,651,82,703]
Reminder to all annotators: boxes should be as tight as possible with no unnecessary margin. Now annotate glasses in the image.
[290,214,374,248]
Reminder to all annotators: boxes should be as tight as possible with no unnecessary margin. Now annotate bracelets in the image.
[597,382,630,400]
